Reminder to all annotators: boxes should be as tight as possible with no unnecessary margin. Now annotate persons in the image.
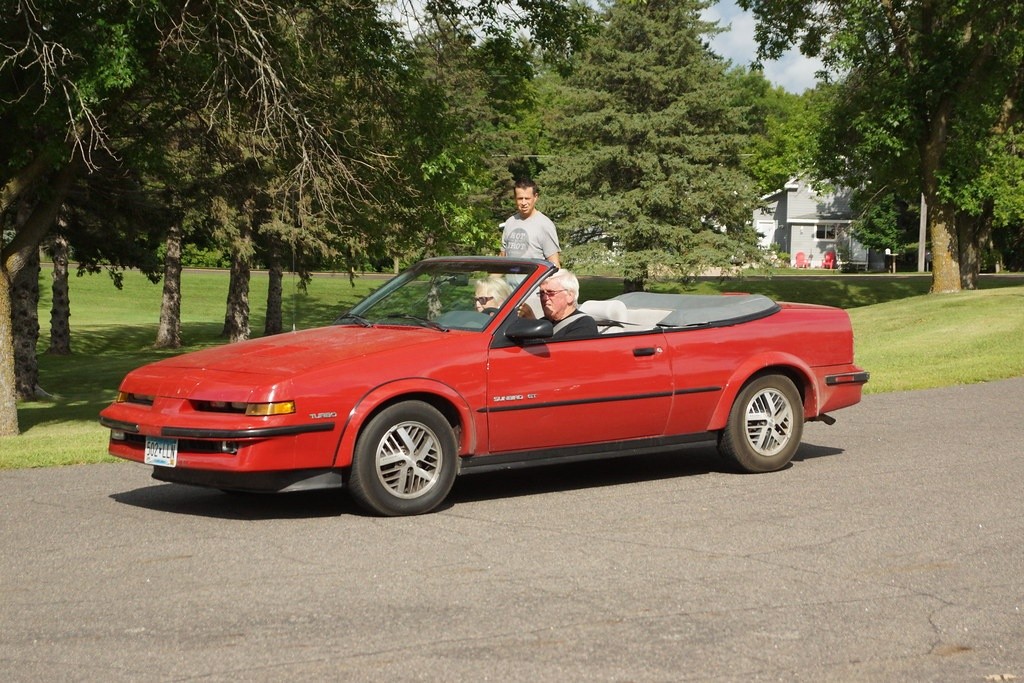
[501,178,562,299]
[516,268,598,338]
[473,277,513,316]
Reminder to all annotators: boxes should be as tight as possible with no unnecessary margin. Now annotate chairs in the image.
[796,251,808,269]
[580,300,630,333]
[822,252,835,268]
[525,286,545,320]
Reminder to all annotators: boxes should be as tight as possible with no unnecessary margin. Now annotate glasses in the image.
[473,296,494,305]
[537,289,567,298]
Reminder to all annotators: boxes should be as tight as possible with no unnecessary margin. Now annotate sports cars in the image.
[98,256,870,517]
